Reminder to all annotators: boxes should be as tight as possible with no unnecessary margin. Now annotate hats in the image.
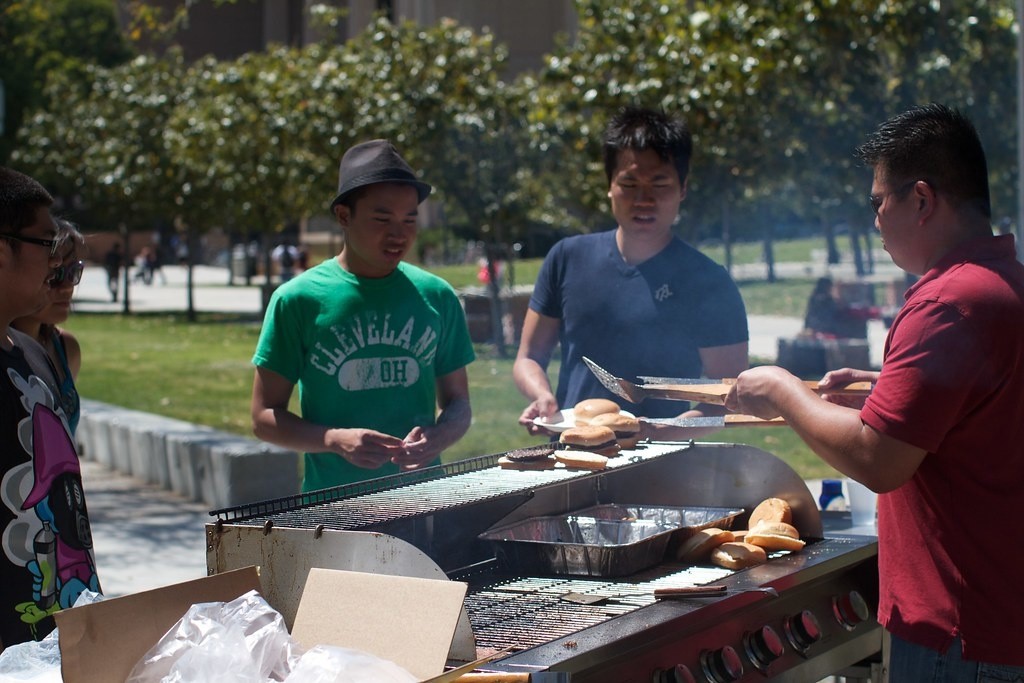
[330,138,432,214]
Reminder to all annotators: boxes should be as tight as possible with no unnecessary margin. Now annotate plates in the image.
[533,408,637,431]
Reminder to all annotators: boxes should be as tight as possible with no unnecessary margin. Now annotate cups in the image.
[843,478,877,528]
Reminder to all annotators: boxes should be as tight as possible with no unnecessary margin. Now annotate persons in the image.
[10,218,83,437]
[273,240,310,274]
[104,243,121,302]
[725,106,1024,683]
[134,233,186,286]
[0,166,103,655]
[252,141,476,506]
[805,278,846,339]
[514,112,750,441]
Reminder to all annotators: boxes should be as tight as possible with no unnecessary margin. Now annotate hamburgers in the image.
[497,398,640,469]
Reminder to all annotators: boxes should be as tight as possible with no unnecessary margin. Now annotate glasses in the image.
[48,263,83,288]
[869,183,935,214]
[0,231,58,256]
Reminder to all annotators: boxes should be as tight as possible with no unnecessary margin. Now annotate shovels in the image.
[582,355,726,412]
[555,586,726,606]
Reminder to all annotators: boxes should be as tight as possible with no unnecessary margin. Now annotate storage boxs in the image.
[52,564,518,683]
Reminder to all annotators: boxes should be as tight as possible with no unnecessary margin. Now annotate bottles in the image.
[818,480,846,510]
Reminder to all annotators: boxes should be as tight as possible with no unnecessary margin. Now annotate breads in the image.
[678,496,807,569]
